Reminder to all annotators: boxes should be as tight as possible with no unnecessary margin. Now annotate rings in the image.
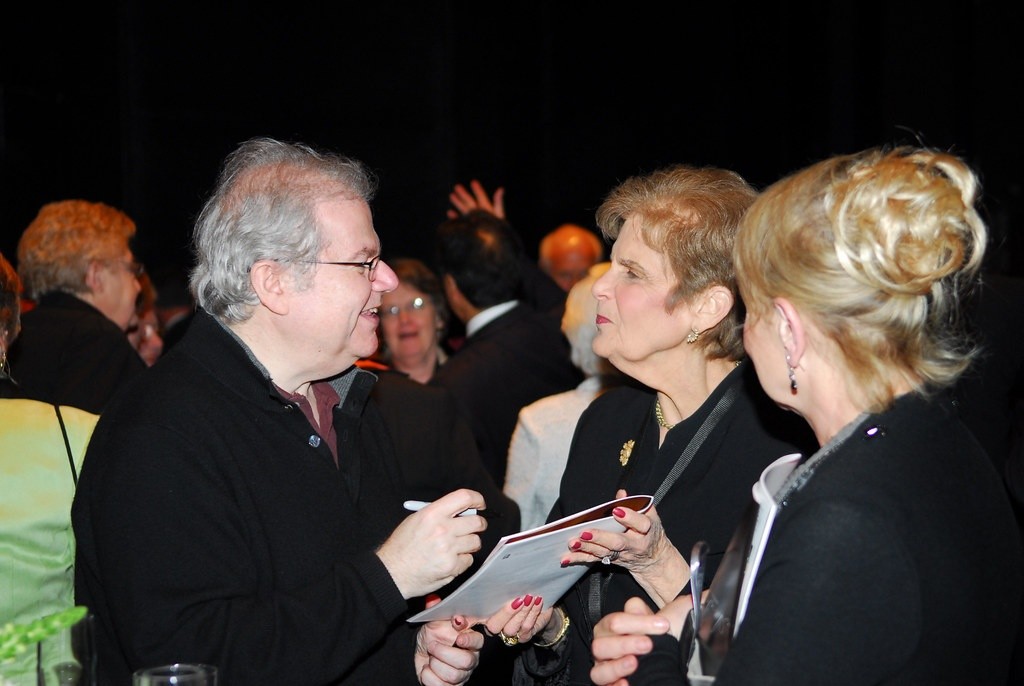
[602,551,619,564]
[500,631,520,646]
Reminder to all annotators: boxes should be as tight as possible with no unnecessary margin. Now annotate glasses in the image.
[379,298,434,316]
[246,255,380,282]
[101,261,143,276]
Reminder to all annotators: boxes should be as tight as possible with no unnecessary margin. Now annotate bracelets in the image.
[533,606,570,647]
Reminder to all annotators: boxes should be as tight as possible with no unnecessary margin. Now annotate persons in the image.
[71,139,488,686]
[484,168,820,686]
[2,200,182,686]
[358,179,631,531]
[589,148,1024,685]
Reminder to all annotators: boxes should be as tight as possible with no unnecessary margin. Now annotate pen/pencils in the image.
[401,500,506,518]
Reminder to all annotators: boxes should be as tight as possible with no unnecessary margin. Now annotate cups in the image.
[132,662,219,686]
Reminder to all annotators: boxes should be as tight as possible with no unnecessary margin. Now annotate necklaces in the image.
[656,401,674,429]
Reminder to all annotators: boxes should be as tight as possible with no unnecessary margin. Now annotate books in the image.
[687,453,802,686]
[406,494,655,623]
